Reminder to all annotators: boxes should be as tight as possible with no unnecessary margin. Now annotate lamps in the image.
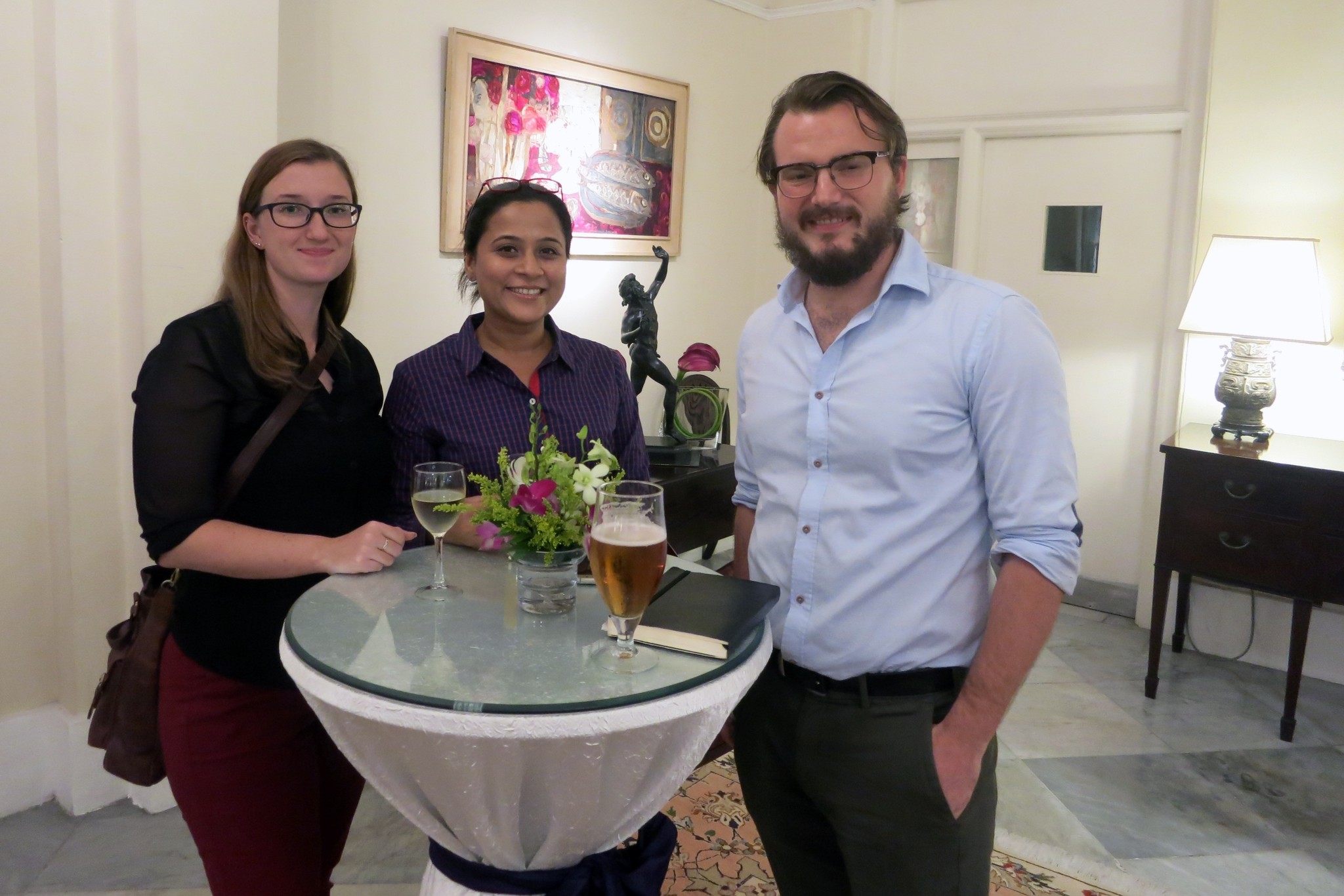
[1178,232,1329,443]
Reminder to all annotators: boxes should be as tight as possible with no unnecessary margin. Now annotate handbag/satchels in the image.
[88,566,178,786]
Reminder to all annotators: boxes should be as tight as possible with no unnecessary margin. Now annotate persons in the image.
[725,70,1083,896]
[618,244,688,445]
[134,139,418,896]
[382,173,666,550]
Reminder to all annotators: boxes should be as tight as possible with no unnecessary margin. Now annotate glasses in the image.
[770,151,892,199]
[252,202,363,228]
[464,177,563,239]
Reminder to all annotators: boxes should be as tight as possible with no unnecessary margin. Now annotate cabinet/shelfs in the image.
[1146,420,1344,744]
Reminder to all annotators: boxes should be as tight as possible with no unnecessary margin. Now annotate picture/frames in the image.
[441,26,690,258]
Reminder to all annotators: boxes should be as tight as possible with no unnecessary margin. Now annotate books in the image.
[606,566,781,659]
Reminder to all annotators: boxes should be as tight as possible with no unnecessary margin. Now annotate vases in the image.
[670,386,729,448]
[512,539,588,615]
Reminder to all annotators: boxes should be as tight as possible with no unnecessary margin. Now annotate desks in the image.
[279,543,774,896]
[644,445,745,556]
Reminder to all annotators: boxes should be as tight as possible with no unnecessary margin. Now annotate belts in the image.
[769,652,954,697]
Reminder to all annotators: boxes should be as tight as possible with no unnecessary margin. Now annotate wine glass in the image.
[587,479,668,672]
[409,461,467,601]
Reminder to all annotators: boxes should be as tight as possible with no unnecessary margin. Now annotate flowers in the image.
[429,397,622,566]
[676,341,722,439]
